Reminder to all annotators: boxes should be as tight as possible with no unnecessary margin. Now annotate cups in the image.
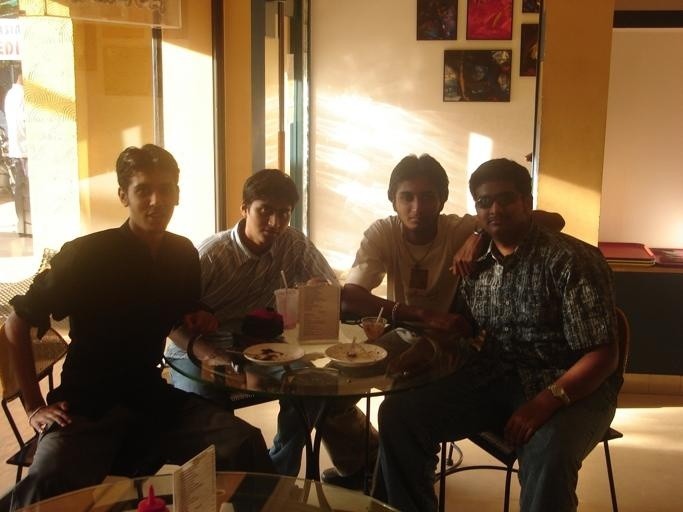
[360,315,385,338]
[274,288,298,328]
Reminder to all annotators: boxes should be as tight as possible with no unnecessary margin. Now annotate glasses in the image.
[476,192,515,208]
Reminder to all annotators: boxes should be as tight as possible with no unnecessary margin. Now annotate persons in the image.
[3,65,34,239]
[377,157,619,512]
[1,142,280,510]
[320,153,566,493]
[168,168,342,479]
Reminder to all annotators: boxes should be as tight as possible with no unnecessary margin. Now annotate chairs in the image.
[439,307,632,511]
[0,317,69,483]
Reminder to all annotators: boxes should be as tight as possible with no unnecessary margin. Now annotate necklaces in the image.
[401,239,432,270]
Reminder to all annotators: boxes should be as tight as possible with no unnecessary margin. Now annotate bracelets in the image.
[28,403,46,426]
[473,223,491,243]
[391,301,402,321]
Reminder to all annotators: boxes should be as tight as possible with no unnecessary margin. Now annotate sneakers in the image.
[322,468,358,488]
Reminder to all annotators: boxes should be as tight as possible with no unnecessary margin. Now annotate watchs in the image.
[547,380,572,407]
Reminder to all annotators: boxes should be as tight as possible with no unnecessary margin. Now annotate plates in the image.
[323,341,388,372]
[244,343,306,368]
